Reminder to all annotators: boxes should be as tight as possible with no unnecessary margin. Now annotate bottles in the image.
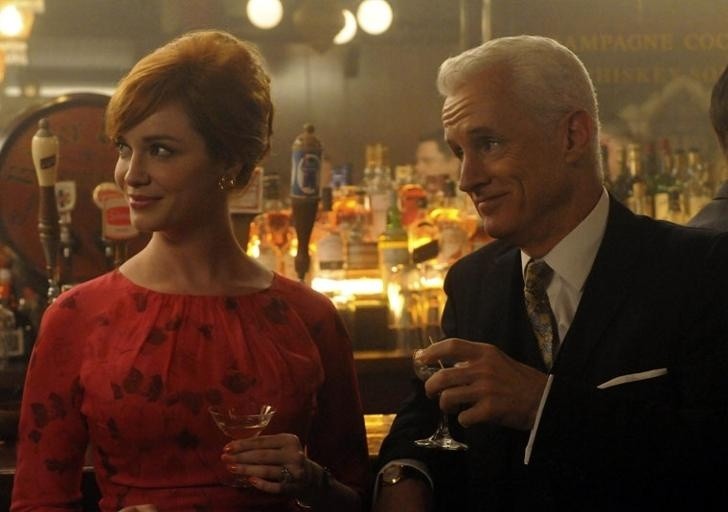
[241,146,491,349]
[0,268,40,365]
[599,142,725,226]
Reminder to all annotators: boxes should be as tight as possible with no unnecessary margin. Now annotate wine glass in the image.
[205,402,279,490]
[410,346,471,453]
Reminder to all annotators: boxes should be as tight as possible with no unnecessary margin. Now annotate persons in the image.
[12,25,372,511]
[683,70,728,234]
[372,32,727,509]
[413,127,460,198]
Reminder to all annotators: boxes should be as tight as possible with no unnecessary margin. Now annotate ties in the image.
[523,261,553,374]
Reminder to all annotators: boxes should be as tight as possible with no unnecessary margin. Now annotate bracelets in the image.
[295,466,335,511]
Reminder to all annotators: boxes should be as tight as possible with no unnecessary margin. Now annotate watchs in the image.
[377,461,428,486]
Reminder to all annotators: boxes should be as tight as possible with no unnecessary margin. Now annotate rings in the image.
[280,463,294,483]
